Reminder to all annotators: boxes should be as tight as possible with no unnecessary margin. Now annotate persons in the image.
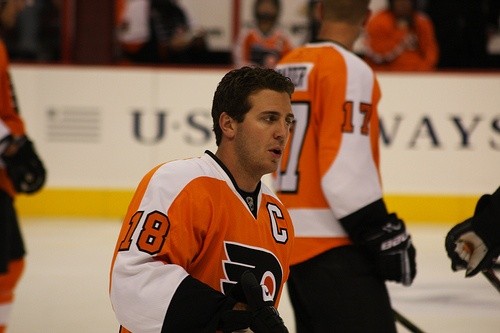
[0,0,46,333]
[366,0,438,71]
[108,67,296,333]
[235,0,295,66]
[270,0,417,333]
[130,0,226,65]
[444,185,500,276]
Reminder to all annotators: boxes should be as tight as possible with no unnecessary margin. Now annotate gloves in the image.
[445,190,500,278]
[1,137,47,194]
[220,273,287,332]
[364,211,417,284]
